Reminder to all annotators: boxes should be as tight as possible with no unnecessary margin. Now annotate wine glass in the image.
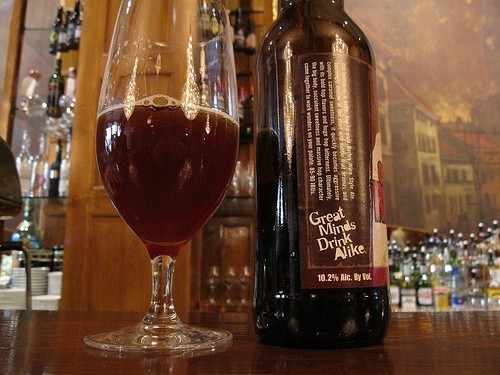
[84,1,240,353]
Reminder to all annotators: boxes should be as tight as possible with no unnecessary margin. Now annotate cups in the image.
[0,259,62,309]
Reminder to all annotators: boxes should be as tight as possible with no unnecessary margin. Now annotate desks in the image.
[0,307,500,375]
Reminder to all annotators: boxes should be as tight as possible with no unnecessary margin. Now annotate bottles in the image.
[16,51,78,119]
[11,198,64,272]
[252,0,391,346]
[388,219,500,312]
[15,126,73,196]
[48,0,83,55]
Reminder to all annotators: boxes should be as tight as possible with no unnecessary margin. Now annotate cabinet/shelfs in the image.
[10,0,277,313]
